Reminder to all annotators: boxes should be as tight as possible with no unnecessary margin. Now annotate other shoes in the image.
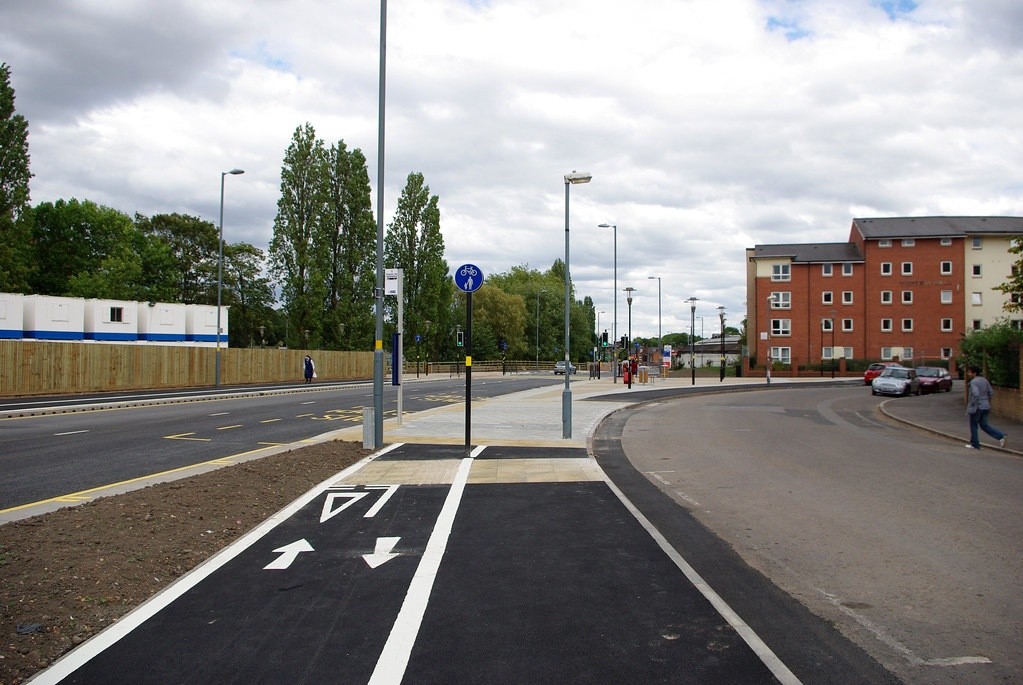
[304,382,312,384]
[1000,434,1008,448]
[965,445,979,449]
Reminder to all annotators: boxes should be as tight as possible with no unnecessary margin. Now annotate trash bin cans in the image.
[639,366,648,385]
[661,365,668,379]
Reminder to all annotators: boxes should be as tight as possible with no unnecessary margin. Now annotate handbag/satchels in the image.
[312,372,318,378]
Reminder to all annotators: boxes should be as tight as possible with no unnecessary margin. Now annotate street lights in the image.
[687,297,700,386]
[536,291,548,369]
[562,172,594,438]
[622,287,637,356]
[716,306,728,382]
[598,223,618,383]
[215,168,245,389]
[597,311,605,361]
[648,277,661,352]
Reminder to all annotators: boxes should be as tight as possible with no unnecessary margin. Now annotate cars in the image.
[554,361,576,375]
[871,367,921,397]
[916,366,953,395]
[864,362,902,385]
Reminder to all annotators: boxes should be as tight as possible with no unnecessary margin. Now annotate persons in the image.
[302,355,315,383]
[964,365,1008,449]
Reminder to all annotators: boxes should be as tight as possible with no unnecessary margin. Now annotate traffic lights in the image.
[602,333,608,347]
[457,332,464,346]
